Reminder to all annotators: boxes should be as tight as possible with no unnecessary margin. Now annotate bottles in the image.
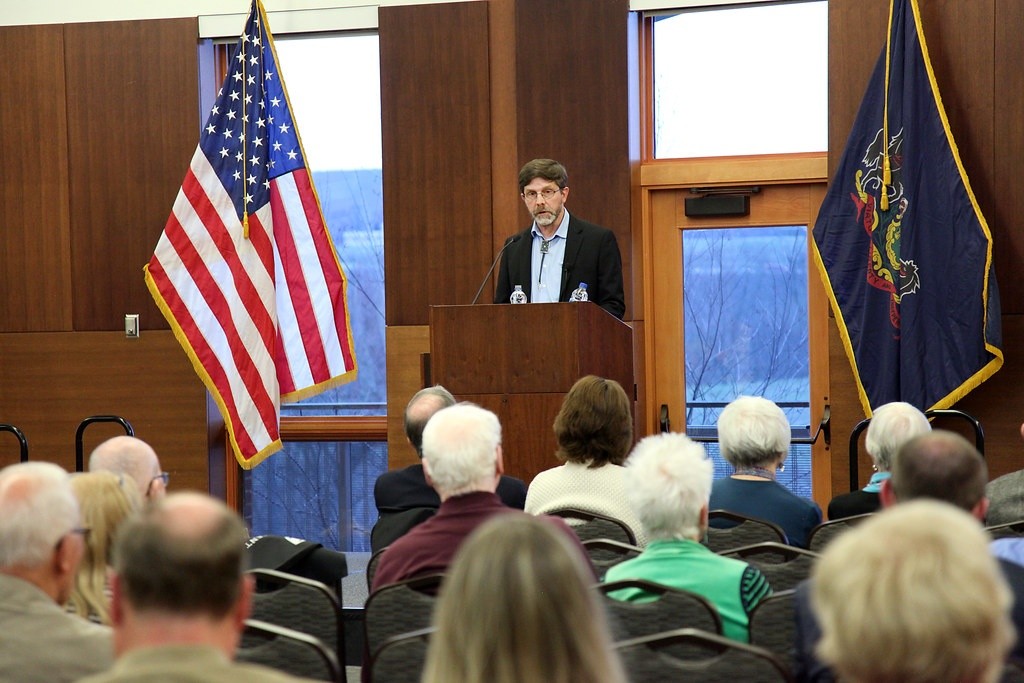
[510,286,527,303]
[569,283,588,302]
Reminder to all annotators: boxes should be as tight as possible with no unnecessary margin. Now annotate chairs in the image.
[543,506,637,561]
[234,617,348,683]
[744,587,802,674]
[615,626,796,683]
[717,540,820,592]
[804,511,876,554]
[362,571,447,662]
[245,534,348,612]
[581,537,644,582]
[245,567,346,668]
[596,578,725,661]
[707,509,791,564]
[362,625,438,683]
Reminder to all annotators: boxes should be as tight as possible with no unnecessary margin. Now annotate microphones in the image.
[472,234,522,304]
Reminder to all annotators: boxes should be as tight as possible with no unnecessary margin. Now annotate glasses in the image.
[146,470,171,497]
[56,523,91,550]
[523,188,562,201]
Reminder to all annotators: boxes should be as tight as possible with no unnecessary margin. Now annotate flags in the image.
[812,0,1003,422]
[142,0,358,471]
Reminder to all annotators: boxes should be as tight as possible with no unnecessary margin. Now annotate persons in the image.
[524,375,648,550]
[421,510,629,683]
[827,402,932,521]
[605,432,775,645]
[371,401,597,594]
[75,490,329,683]
[493,158,626,320]
[985,422,1024,568]
[809,499,1017,683]
[0,461,115,683]
[708,396,823,561]
[374,386,527,516]
[88,435,165,502]
[795,430,1024,683]
[59,470,142,627]
